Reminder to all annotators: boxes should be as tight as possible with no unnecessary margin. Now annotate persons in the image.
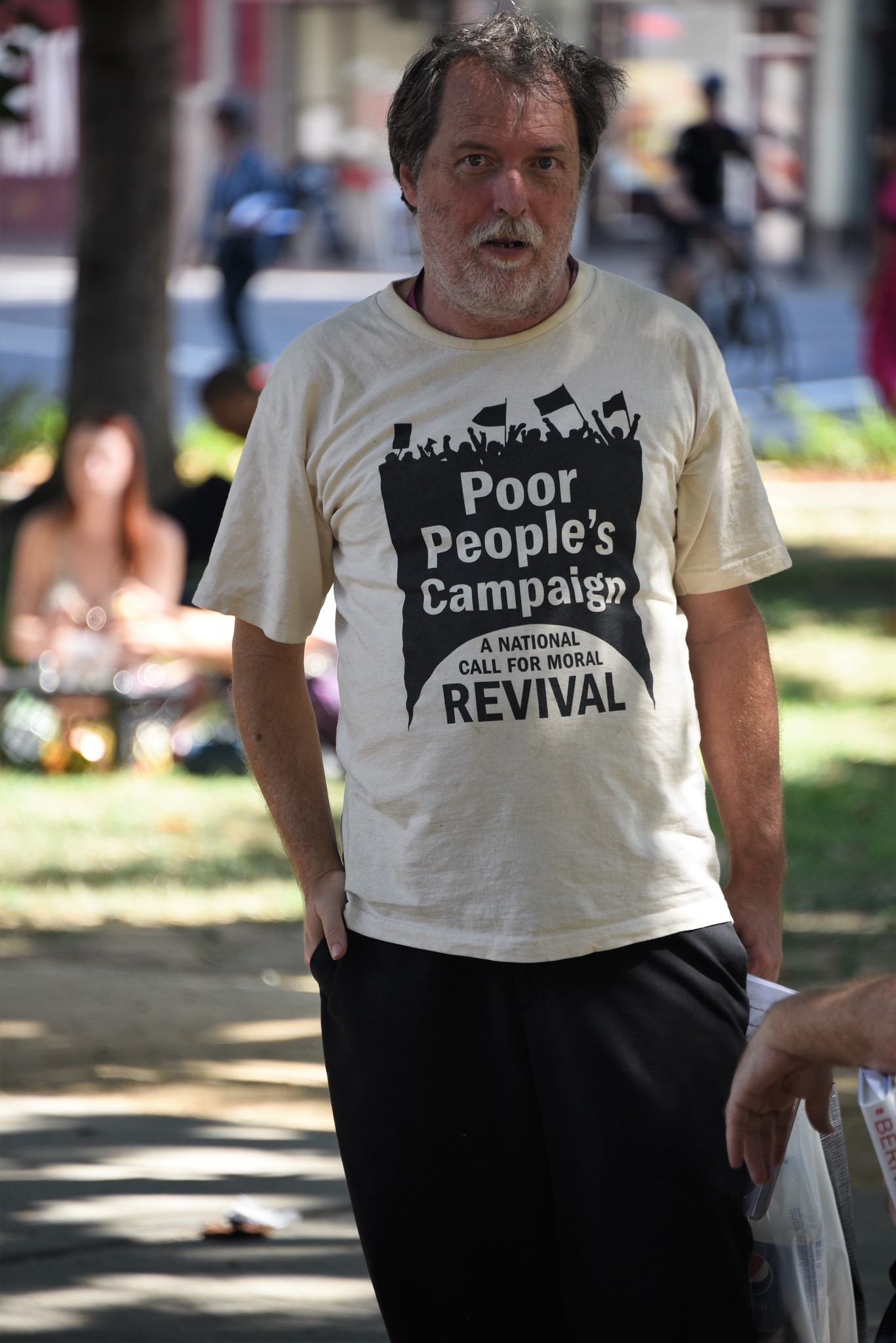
[189,3,794,1342]
[0,406,185,772]
[726,968,896,1343]
[673,71,760,342]
[204,94,276,369]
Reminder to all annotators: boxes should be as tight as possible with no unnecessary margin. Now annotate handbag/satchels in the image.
[750,1100,858,1343]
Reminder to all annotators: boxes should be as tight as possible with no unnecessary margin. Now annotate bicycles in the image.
[661,214,785,363]
